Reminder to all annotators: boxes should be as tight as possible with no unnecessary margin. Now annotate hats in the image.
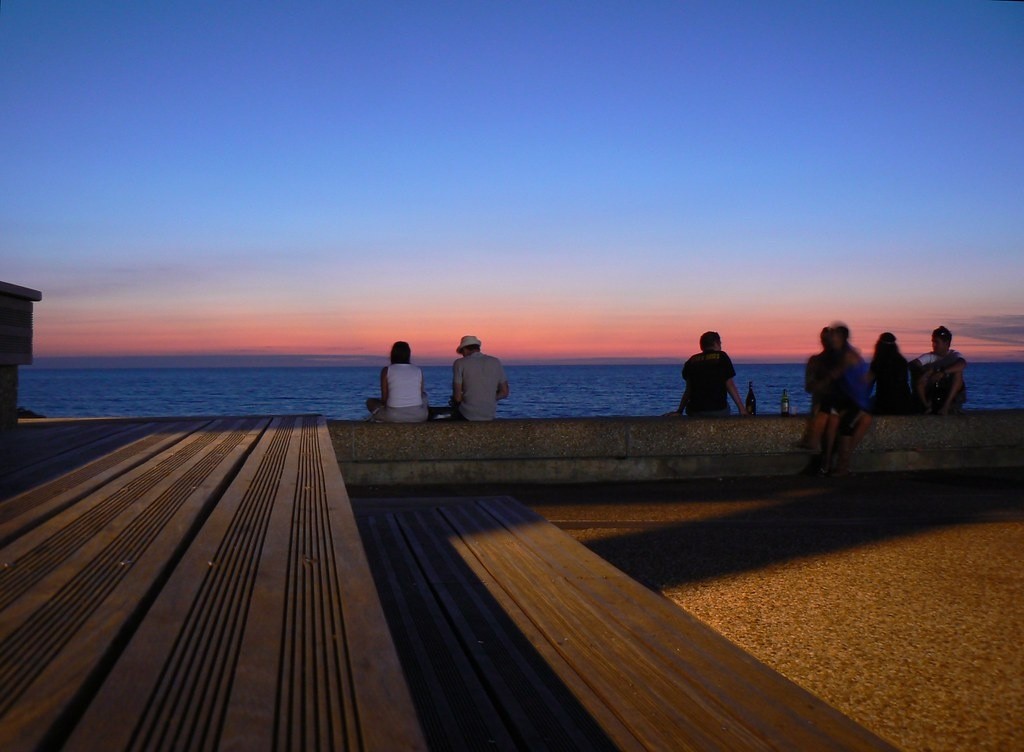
[456,335,482,353]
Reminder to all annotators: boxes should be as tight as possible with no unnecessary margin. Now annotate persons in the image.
[663,331,748,416]
[367,341,428,423]
[865,331,917,416]
[801,326,872,478]
[907,326,966,415]
[448,335,509,421]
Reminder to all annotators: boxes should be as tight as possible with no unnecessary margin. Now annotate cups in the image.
[790,406,796,416]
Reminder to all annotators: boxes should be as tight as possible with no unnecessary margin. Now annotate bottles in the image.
[746,382,756,414]
[782,389,789,417]
